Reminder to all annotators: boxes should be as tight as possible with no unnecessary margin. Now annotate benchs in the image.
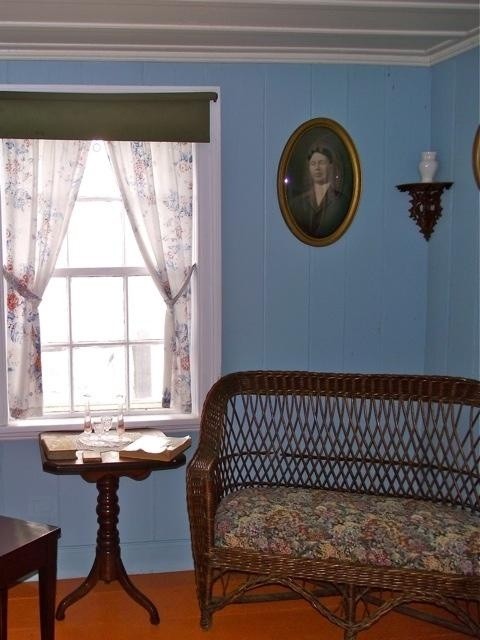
[184,370,479,638]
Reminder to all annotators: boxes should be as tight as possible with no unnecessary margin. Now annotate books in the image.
[40,435,192,462]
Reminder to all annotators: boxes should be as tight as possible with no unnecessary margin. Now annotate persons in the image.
[289,146,352,238]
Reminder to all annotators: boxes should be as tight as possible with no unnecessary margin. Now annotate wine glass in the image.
[93,416,112,440]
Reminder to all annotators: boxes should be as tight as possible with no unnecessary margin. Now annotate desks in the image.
[37,427,188,625]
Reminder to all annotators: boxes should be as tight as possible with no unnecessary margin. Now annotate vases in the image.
[418,151,438,183]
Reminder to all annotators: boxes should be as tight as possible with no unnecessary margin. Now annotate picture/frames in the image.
[276,119,361,248]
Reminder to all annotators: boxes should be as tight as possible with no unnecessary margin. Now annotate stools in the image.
[0,515,62,640]
